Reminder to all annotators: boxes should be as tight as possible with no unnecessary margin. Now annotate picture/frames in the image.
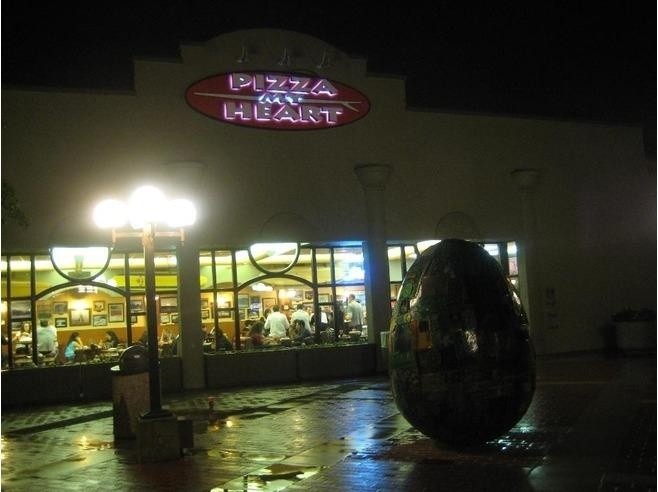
[160,295,232,324]
[237,293,276,320]
[53,299,146,326]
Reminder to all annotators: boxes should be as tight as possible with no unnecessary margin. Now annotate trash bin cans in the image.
[111,346,162,438]
[380,331,390,375]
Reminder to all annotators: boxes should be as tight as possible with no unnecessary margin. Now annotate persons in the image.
[139,326,233,361]
[10,319,59,362]
[64,331,120,361]
[240,294,362,350]
